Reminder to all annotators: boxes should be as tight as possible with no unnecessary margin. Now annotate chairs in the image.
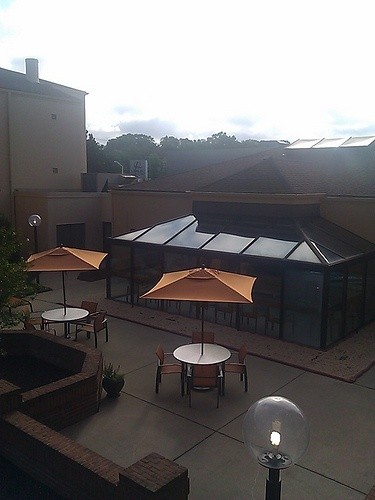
[186,365,221,407]
[23,299,56,335]
[68,301,108,348]
[156,344,185,395]
[193,331,214,343]
[223,345,248,394]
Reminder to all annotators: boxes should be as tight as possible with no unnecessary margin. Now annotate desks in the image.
[173,342,232,391]
[42,308,89,339]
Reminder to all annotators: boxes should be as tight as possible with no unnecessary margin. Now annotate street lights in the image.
[244,395,307,500]
[27,214,42,286]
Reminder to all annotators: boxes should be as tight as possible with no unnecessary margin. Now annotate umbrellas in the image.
[21,244,108,337]
[140,264,257,356]
